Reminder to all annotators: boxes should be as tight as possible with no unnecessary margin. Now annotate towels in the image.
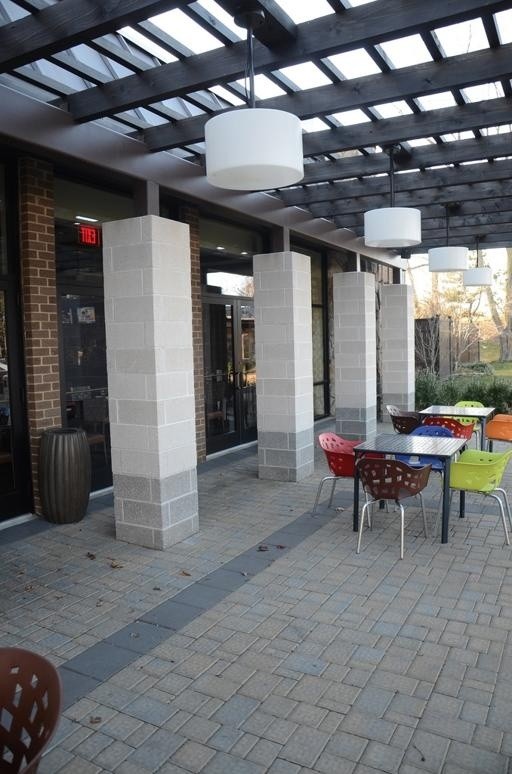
[38,426,93,524]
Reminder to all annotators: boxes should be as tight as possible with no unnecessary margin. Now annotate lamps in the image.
[205,383,225,438]
[390,425,454,521]
[485,413,512,461]
[385,404,427,434]
[1,647,60,772]
[442,400,485,450]
[311,431,385,528]
[423,418,475,450]
[356,458,433,559]
[79,398,110,472]
[435,446,512,546]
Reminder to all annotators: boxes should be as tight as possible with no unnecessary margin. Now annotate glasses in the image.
[363,143,423,249]
[463,234,493,286]
[428,202,468,272]
[204,9,307,191]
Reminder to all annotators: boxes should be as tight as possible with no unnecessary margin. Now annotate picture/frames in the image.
[353,433,468,544]
[418,405,496,461]
